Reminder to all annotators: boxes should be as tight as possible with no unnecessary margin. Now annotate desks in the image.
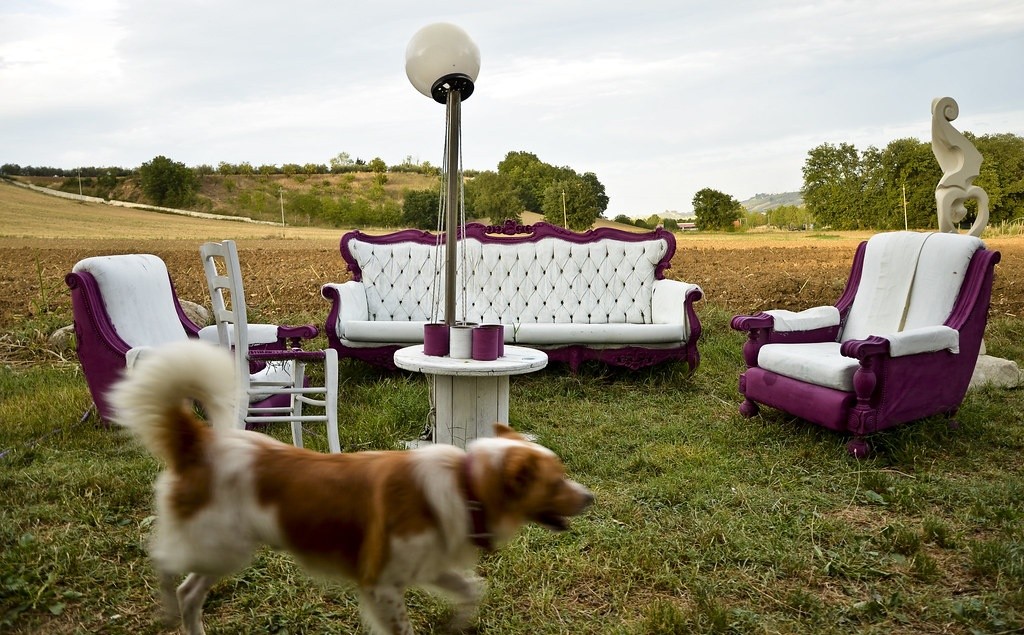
[394,342,551,460]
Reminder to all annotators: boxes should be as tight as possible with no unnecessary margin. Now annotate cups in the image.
[480,324,504,356]
[450,326,472,359]
[424,324,449,356]
[472,328,499,362]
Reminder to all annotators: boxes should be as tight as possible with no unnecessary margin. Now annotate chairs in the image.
[200,241,344,458]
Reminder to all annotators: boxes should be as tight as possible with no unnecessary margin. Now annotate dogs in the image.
[106,337,596,635]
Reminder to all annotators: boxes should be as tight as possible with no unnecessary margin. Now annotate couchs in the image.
[728,230,1001,459]
[66,251,309,432]
[321,222,705,373]
